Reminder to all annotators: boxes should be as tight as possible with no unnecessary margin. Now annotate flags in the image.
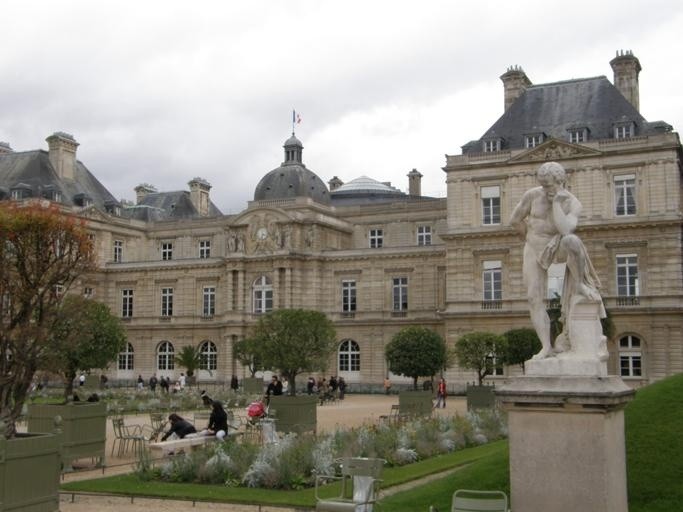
[292,110,301,124]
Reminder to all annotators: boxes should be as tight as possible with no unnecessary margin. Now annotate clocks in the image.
[255,227,268,241]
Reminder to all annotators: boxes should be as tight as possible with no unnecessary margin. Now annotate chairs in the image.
[314,456,386,512]
[378,405,401,431]
[111,408,249,459]
[428,488,509,512]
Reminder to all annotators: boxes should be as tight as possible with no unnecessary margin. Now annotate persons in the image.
[509,161,602,358]
[79,373,85,386]
[184,400,228,443]
[136,371,184,395]
[159,413,196,440]
[436,377,447,409]
[383,377,392,394]
[100,375,107,387]
[265,375,348,407]
[230,374,239,393]
[200,389,214,406]
